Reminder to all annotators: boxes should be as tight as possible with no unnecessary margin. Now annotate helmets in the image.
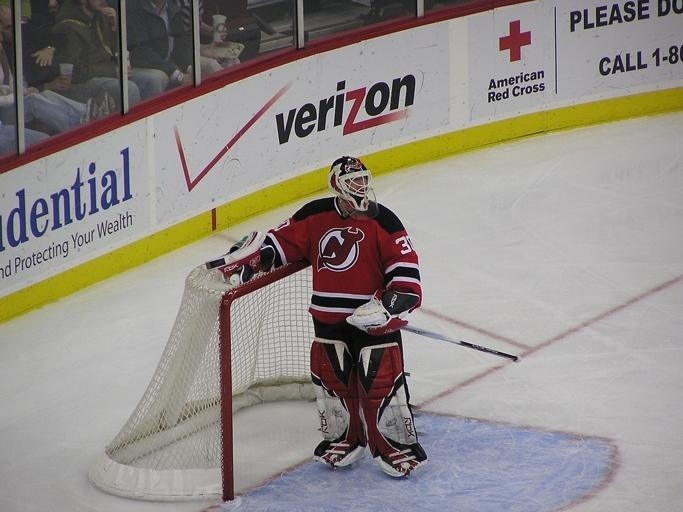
[329,156,376,211]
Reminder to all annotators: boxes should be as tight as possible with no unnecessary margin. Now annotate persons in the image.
[219,156,427,477]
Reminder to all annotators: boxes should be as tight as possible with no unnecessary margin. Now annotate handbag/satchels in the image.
[227,17,260,42]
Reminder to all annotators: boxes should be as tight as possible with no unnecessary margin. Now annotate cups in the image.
[59,63,73,77]
[211,15,227,43]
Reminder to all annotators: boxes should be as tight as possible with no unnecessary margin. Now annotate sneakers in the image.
[84,92,116,123]
